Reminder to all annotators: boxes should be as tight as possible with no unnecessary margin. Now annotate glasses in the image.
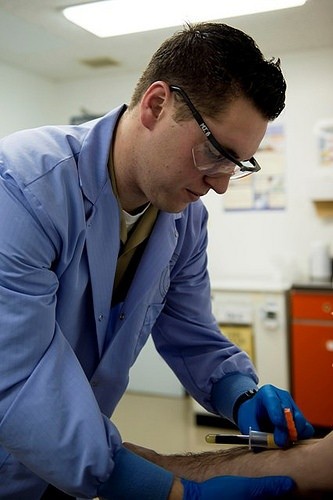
[168,83,261,180]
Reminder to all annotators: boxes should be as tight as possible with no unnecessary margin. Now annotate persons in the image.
[0,24,320,500]
[120,431,333,500]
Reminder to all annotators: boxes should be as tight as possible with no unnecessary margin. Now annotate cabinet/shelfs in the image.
[285,285,333,428]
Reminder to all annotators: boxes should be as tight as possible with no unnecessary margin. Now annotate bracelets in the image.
[231,388,260,426]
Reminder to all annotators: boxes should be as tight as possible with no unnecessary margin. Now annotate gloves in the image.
[237,384,314,454]
[180,474,293,500]
